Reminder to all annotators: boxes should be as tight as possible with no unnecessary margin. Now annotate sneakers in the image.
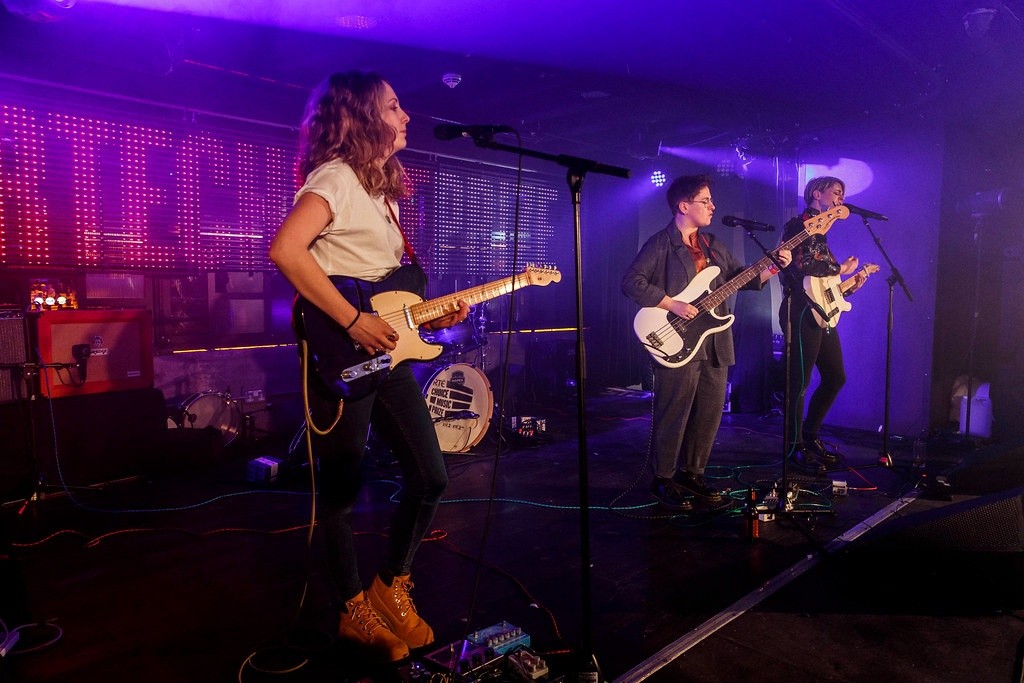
[367,572,435,648]
[339,589,409,661]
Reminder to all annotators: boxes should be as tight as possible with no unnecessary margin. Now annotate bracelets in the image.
[768,265,779,274]
[346,310,360,330]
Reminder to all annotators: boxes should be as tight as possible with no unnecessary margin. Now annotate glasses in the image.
[685,198,714,208]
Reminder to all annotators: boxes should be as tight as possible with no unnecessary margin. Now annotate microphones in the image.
[73,344,90,380]
[843,203,888,220]
[721,216,776,232]
[433,123,512,140]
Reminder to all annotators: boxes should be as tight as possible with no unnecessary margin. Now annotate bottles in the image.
[744,488,760,542]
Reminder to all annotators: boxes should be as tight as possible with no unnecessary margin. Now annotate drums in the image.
[411,358,495,453]
[166,391,242,450]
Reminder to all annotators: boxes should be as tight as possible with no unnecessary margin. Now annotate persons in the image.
[620,175,792,513]
[270,69,470,663]
[776,177,867,473]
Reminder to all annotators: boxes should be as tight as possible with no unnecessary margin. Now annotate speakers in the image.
[34,310,153,400]
[0,305,32,402]
[945,448,990,493]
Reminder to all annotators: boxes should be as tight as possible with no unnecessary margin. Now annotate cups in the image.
[911,441,926,468]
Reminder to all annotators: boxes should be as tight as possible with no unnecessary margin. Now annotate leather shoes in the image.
[673,469,721,502]
[649,476,692,508]
[806,437,839,464]
[790,447,827,474]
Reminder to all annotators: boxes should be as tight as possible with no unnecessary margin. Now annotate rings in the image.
[376,347,382,352]
[687,314,690,317]
[388,331,396,339]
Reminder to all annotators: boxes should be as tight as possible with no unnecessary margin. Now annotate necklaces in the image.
[385,203,391,223]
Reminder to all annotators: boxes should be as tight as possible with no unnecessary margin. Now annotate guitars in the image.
[292,261,561,408]
[633,201,850,368]
[803,263,882,331]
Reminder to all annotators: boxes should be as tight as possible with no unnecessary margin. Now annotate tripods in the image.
[817,216,918,482]
[746,229,834,554]
